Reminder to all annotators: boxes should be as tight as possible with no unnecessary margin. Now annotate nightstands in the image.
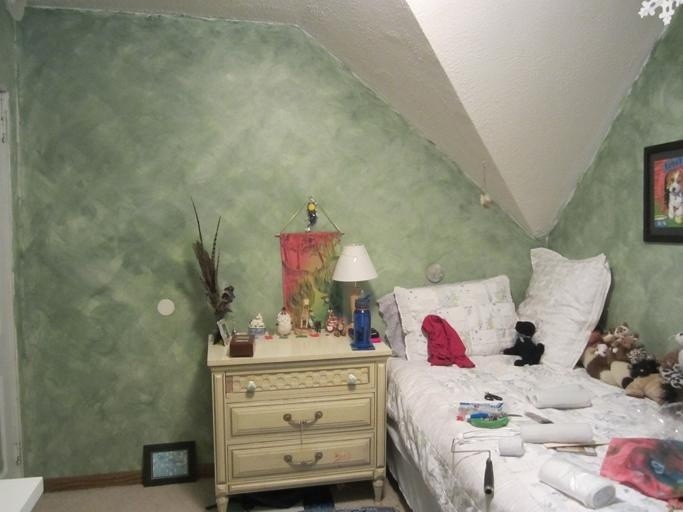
[207,323,394,512]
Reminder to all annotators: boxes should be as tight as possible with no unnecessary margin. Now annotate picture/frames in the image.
[141,441,195,488]
[641,138,681,242]
[215,320,233,345]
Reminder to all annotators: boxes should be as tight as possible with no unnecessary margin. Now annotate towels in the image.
[526,384,593,411]
[516,423,597,445]
[540,455,616,508]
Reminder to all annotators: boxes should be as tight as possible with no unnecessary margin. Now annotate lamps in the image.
[331,241,379,342]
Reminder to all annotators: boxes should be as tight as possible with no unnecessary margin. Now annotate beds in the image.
[378,296,682,511]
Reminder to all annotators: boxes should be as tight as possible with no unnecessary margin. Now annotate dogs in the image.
[665,168,683,219]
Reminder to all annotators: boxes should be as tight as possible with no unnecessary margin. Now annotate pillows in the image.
[387,269,518,361]
[373,293,407,362]
[513,246,612,375]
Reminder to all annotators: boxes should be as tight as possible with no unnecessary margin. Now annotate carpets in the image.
[202,477,412,512]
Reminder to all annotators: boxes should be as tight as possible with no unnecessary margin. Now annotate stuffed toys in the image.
[506,319,683,405]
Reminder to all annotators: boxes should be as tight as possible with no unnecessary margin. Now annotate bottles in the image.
[352,292,373,348]
